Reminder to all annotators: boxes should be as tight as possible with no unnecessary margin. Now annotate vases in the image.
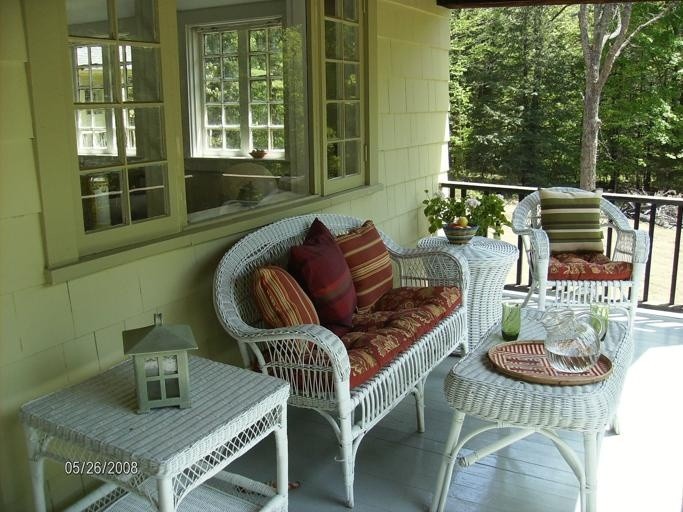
[442,224,479,245]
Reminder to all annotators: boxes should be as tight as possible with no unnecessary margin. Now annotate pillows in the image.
[287,217,357,329]
[248,265,320,353]
[537,187,604,255]
[334,220,393,314]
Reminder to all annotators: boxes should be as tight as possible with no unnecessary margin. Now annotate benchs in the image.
[212,213,470,509]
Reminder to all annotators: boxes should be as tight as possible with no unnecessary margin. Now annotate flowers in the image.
[422,187,515,240]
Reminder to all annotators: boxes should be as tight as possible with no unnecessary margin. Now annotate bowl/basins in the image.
[249,153,267,158]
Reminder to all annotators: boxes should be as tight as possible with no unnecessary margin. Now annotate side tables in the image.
[415,234,519,358]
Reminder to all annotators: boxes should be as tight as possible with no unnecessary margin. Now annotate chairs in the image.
[510,186,650,328]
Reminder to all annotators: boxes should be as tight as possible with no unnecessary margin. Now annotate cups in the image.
[500,301,522,342]
[589,302,610,341]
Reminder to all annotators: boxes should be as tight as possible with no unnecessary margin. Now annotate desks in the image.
[18,351,291,512]
